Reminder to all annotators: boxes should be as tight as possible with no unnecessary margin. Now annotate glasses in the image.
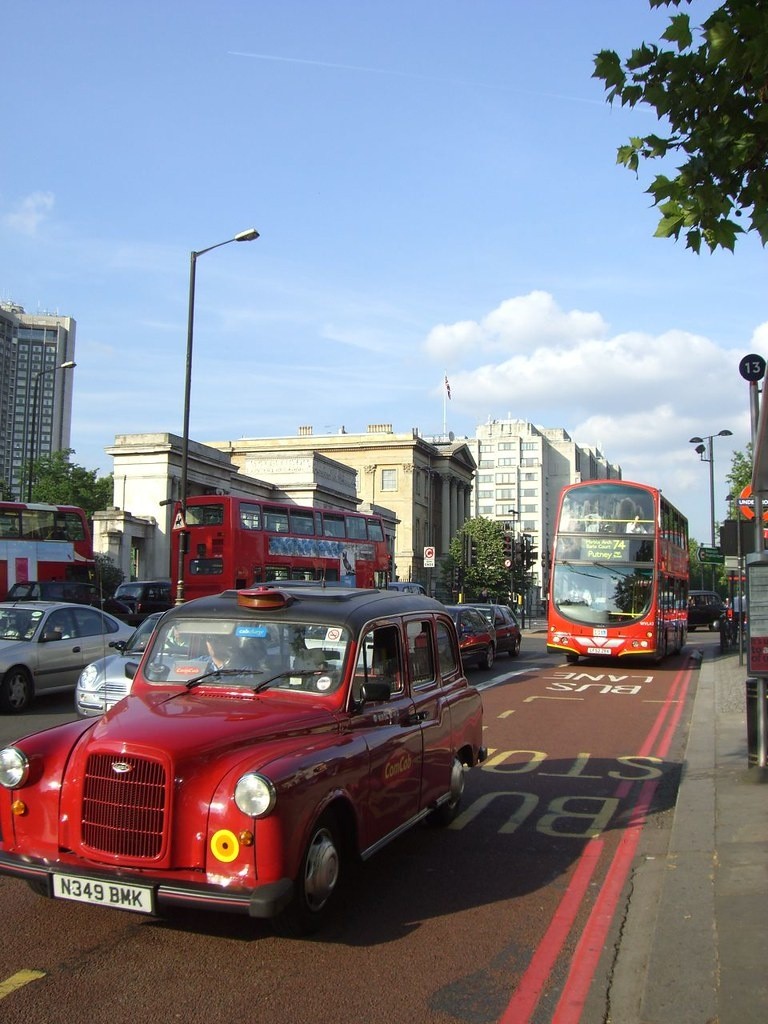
[207,635,225,642]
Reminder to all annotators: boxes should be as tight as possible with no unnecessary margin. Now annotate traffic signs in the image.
[694,547,726,565]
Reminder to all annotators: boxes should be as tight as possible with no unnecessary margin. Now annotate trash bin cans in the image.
[745,678,768,768]
[719,617,739,655]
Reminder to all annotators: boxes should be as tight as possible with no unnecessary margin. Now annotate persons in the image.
[185,633,282,687]
[626,513,647,534]
[165,626,188,649]
[44,613,71,639]
[565,579,592,606]
[584,515,600,533]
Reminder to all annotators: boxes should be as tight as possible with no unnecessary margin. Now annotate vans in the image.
[5,581,134,616]
[387,581,429,596]
[115,581,174,614]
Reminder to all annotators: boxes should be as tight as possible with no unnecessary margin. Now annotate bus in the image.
[171,495,395,608]
[547,481,690,666]
[1,500,102,607]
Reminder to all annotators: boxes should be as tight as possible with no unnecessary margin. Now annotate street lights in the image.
[689,429,734,604]
[159,229,259,605]
[27,361,77,502]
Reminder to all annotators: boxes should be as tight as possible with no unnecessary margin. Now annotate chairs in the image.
[291,650,336,694]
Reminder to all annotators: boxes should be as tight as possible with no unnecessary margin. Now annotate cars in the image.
[442,605,498,673]
[290,620,379,674]
[461,603,523,657]
[729,596,747,623]
[689,590,726,632]
[0,584,488,931]
[74,612,293,721]
[0,601,140,715]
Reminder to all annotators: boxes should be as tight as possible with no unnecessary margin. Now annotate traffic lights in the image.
[516,542,525,566]
[526,540,538,570]
[502,532,513,560]
